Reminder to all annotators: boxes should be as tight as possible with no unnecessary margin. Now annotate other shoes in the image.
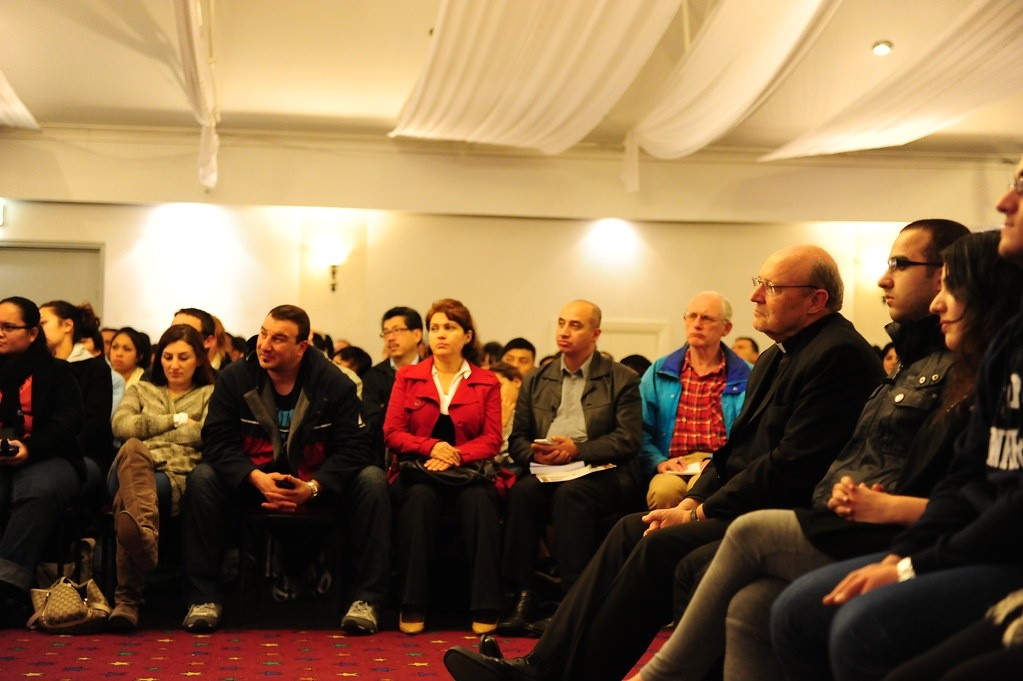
[398,607,424,633]
[472,614,499,634]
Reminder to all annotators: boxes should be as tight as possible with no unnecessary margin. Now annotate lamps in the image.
[309,220,355,292]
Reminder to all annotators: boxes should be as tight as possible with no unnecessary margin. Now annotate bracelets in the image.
[896,556,916,583]
[308,481,318,498]
[690,508,698,521]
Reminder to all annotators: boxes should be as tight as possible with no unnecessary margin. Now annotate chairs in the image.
[55,502,116,595]
[237,508,348,625]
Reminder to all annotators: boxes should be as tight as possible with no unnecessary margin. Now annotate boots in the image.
[498,591,535,630]
[109,534,137,632]
[522,617,551,633]
[115,449,158,570]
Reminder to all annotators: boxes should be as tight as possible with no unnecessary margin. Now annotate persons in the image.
[444,154,1023,681]
[0,296,502,635]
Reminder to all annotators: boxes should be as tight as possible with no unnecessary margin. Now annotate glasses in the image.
[379,328,410,337]
[0,324,31,332]
[751,276,820,295]
[888,258,943,272]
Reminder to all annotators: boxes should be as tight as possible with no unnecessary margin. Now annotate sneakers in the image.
[340,599,377,634]
[182,602,220,629]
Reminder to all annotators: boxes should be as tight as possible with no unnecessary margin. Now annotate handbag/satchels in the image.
[26,576,110,631]
[398,455,497,487]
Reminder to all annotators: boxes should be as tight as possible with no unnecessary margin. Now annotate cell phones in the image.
[534,439,553,445]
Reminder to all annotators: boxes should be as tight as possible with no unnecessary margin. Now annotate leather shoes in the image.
[443,646,538,681]
[479,635,503,658]
